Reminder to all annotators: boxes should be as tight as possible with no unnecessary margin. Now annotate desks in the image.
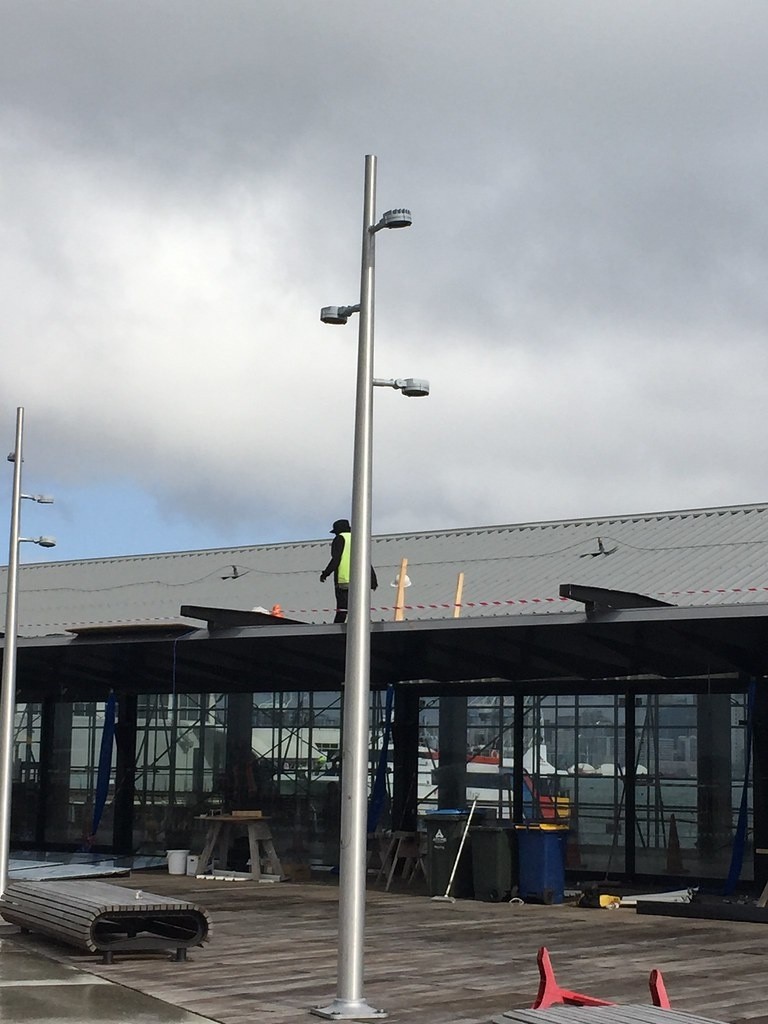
[489,1001,731,1024]
[0,880,216,965]
[192,814,287,883]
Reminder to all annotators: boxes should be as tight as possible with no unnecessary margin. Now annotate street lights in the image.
[319,150,427,1020]
[0,407,59,903]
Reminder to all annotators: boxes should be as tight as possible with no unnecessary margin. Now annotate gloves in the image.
[371,583,378,591]
[320,573,327,583]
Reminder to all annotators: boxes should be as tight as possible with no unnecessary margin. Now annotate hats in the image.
[330,520,351,533]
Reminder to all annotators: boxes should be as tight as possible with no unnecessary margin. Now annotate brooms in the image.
[431,792,480,903]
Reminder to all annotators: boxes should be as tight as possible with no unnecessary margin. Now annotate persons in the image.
[320,518,378,623]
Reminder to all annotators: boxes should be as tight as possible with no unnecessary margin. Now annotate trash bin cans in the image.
[467,816,515,902]
[168,849,188,875]
[512,822,569,904]
[423,808,486,895]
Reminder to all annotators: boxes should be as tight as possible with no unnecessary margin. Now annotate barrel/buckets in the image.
[166,850,190,875]
